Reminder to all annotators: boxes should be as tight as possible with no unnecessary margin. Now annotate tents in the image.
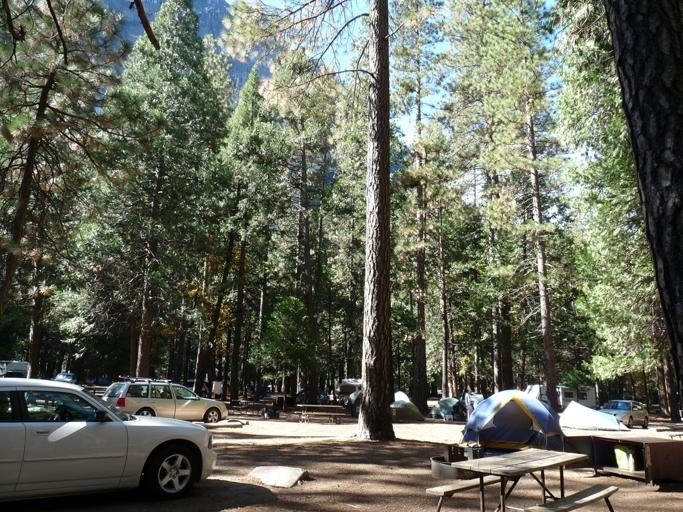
[389,399,425,422]
[426,395,468,422]
[456,388,564,461]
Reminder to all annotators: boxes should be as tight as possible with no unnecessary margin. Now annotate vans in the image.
[556,385,597,409]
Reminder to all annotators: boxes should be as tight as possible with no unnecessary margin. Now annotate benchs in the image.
[294,411,302,422]
[521,484,619,512]
[426,475,501,512]
[302,412,346,424]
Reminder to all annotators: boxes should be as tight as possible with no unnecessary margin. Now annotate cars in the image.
[298,379,361,417]
[0,360,228,501]
[598,399,649,429]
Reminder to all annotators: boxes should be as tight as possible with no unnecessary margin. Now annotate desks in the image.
[297,404,342,411]
[450,448,590,512]
[593,437,683,484]
